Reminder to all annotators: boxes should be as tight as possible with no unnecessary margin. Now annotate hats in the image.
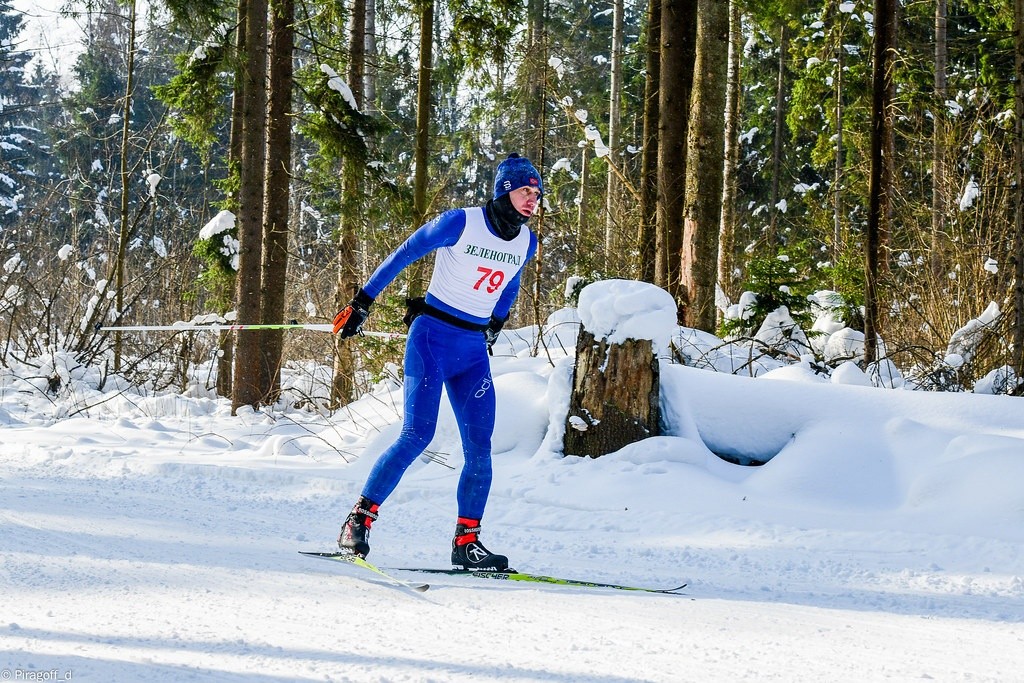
[493,153,544,201]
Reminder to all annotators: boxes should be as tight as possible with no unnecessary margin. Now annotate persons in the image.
[331,151,543,570]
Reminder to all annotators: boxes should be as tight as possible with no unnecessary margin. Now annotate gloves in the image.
[333,294,370,339]
[486,319,504,356]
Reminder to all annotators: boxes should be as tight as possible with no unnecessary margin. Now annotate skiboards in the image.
[300,551,688,609]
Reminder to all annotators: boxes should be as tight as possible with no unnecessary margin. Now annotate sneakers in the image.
[338,513,370,555]
[451,540,509,571]
